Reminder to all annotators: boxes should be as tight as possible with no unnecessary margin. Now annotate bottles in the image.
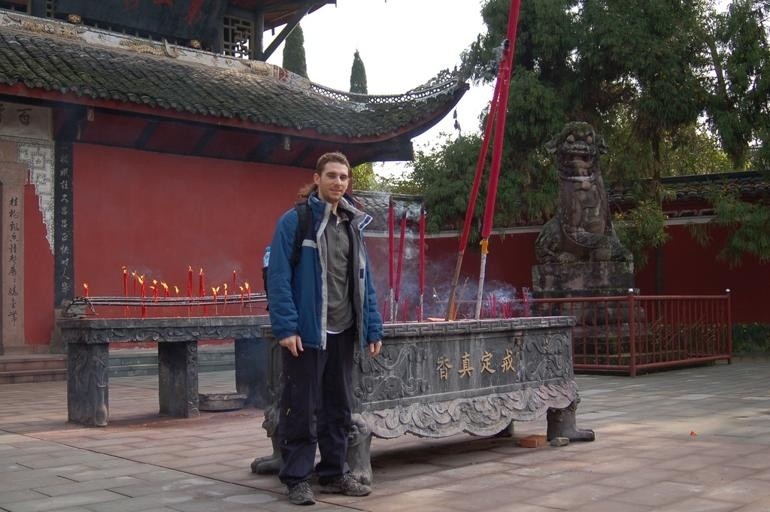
[262,245,272,268]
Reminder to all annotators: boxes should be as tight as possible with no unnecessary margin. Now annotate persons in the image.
[266,153,383,505]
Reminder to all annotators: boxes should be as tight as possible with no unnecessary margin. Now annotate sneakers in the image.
[289,480,316,505]
[319,475,372,496]
